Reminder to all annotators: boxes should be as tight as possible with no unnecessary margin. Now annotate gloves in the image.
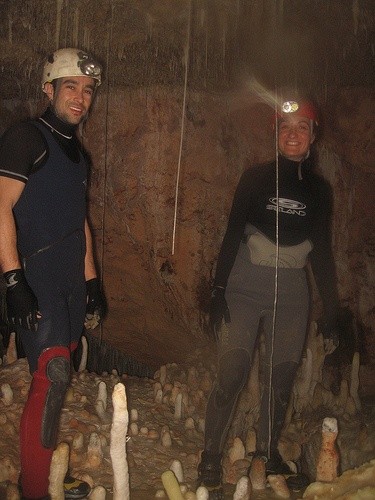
[204,285,230,328]
[321,295,344,331]
[3,268,44,334]
[82,276,106,329]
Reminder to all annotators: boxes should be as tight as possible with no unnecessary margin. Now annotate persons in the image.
[1,48,107,500]
[198,96,343,492]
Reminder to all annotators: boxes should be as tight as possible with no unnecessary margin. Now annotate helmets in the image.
[272,95,321,128]
[41,47,102,87]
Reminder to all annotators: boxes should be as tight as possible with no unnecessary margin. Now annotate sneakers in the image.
[249,452,310,493]
[198,451,224,492]
[16,472,92,500]
